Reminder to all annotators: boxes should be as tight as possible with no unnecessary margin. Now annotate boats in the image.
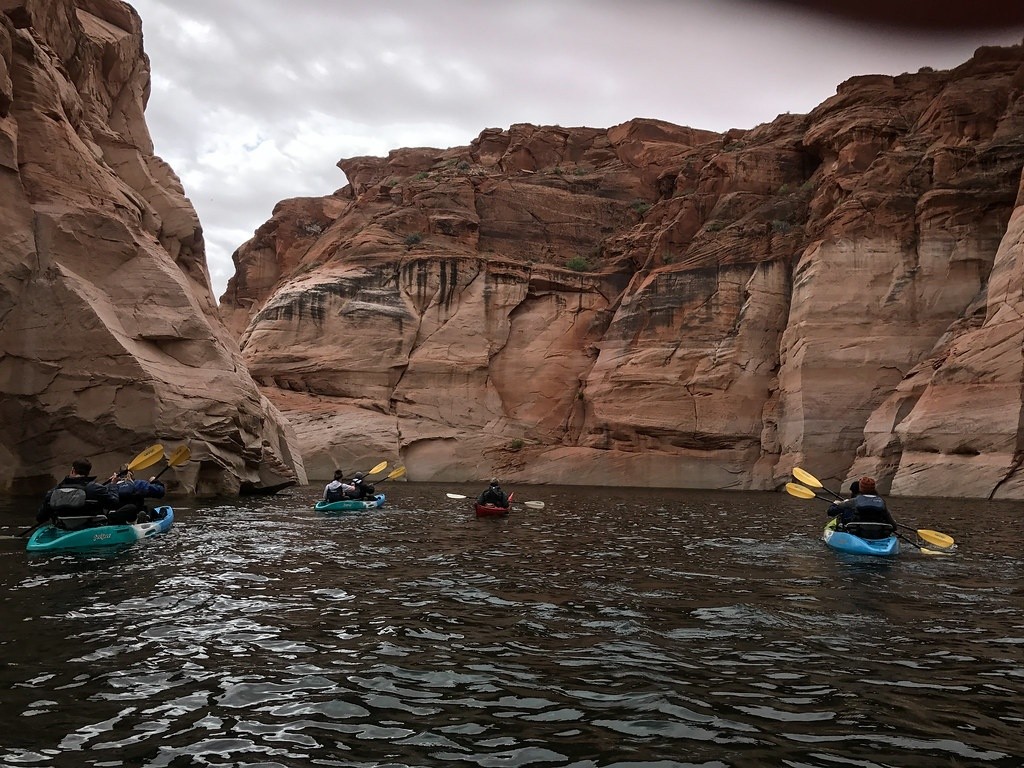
[822,517,902,557]
[474,505,511,517]
[312,493,386,511]
[25,503,176,553]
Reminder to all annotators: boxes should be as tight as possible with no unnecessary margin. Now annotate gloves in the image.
[369,483,373,487]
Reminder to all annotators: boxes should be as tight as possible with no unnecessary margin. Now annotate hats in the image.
[491,477,499,486]
[859,477,876,494]
[335,469,343,479]
[849,481,859,491]
[355,472,362,478]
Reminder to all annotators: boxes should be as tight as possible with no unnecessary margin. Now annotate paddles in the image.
[446,493,545,509]
[354,460,388,483]
[786,483,955,549]
[101,443,164,487]
[373,465,406,486]
[155,444,192,480]
[792,466,931,553]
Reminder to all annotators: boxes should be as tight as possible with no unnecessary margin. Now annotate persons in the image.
[827,476,898,540]
[345,472,378,501]
[476,477,510,508]
[322,469,355,504]
[36,457,166,531]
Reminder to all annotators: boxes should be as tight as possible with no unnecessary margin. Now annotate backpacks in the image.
[484,486,501,503]
